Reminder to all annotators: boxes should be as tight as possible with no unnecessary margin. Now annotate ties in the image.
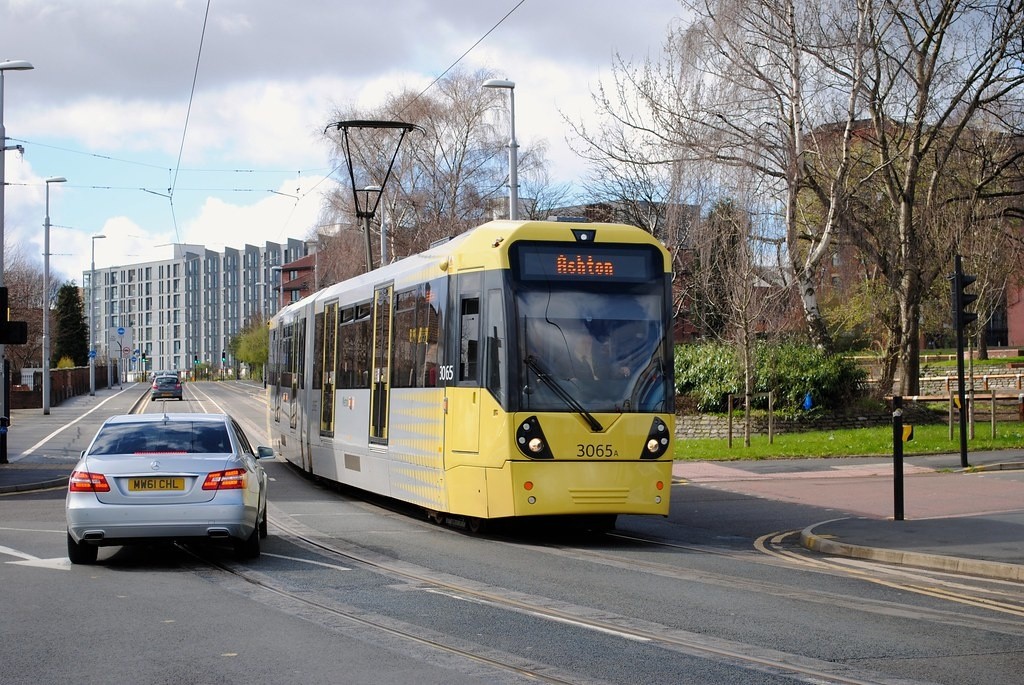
[581,355,594,381]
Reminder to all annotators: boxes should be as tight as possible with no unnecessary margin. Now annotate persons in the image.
[557,330,631,380]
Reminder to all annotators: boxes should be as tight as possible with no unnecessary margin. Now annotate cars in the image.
[65,413,275,564]
[151,375,183,401]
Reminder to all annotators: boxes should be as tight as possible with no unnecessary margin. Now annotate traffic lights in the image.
[222,352,225,361]
[195,356,197,363]
[142,353,145,362]
[955,255,979,331]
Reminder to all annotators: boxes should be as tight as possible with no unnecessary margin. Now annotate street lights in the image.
[364,186,386,266]
[481,79,518,221]
[302,238,319,292]
[88,235,107,397]
[0,59,36,417]
[42,177,67,415]
[107,265,120,389]
[271,265,283,310]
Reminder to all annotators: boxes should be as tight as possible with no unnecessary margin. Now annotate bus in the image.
[263,216,675,532]
